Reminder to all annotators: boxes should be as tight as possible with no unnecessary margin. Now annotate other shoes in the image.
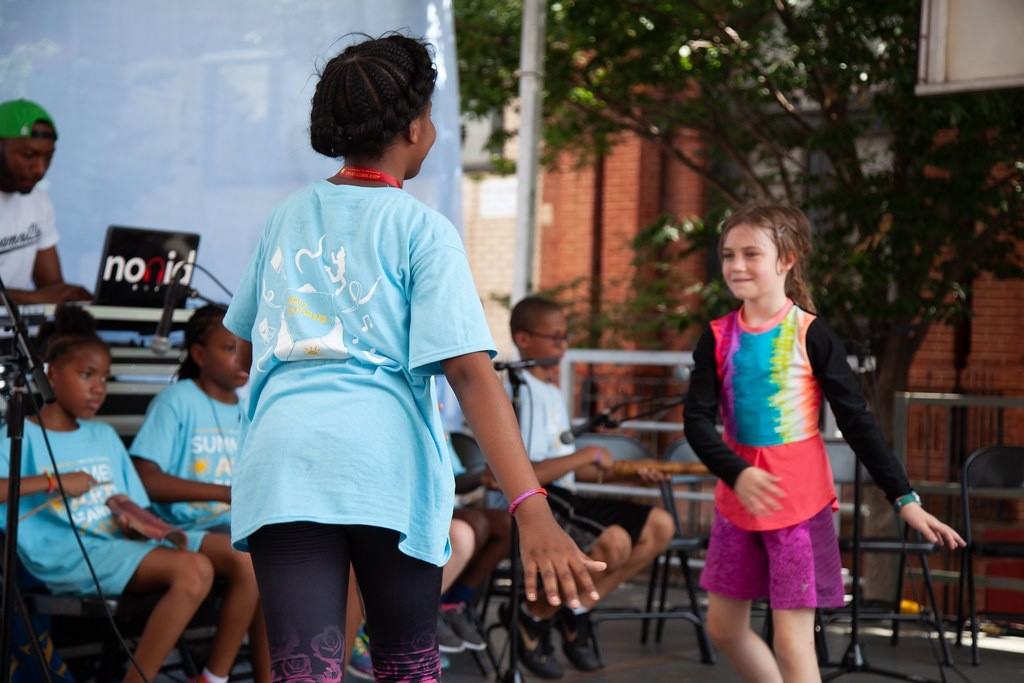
[187,672,211,683]
[347,624,375,680]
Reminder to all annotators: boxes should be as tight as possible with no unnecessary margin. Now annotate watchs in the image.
[895,490,921,512]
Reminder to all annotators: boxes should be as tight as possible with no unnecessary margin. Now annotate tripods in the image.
[820,336,942,683]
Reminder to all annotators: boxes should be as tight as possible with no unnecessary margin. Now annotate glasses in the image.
[529,331,572,344]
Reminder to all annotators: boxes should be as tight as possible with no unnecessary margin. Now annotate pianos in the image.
[0,304,195,374]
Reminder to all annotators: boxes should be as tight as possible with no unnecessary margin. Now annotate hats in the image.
[0,99,58,139]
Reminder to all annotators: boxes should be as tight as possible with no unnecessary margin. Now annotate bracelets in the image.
[44,469,57,494]
[509,487,549,515]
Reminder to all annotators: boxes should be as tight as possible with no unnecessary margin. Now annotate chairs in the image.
[0,445,1024,683]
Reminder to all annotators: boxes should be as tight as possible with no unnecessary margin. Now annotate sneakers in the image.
[437,609,486,654]
[554,601,599,672]
[503,604,565,678]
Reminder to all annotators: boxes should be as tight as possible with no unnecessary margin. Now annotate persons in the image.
[221,26,607,683]
[681,194,966,683]
[0,98,94,304]
[129,296,676,682]
[1,328,259,683]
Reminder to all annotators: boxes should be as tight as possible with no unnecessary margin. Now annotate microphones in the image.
[561,406,618,443]
[153,268,186,356]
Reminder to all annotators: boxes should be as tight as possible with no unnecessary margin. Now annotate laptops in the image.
[74,226,202,307]
